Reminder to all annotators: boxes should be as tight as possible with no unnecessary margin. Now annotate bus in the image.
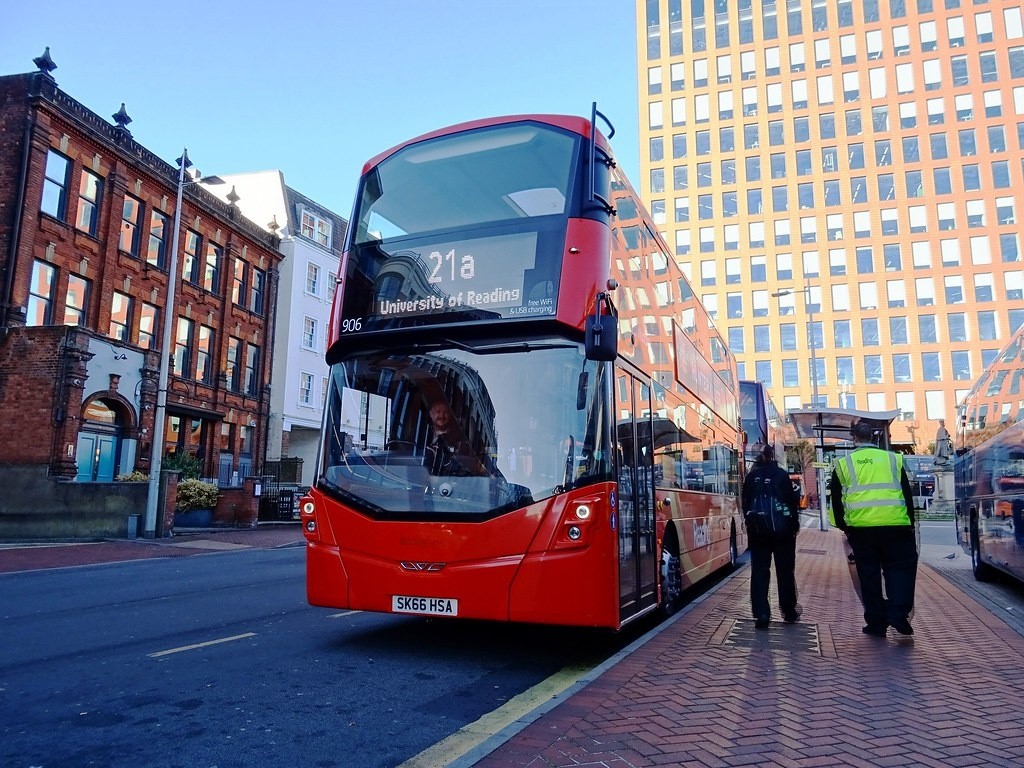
[739,380,795,483]
[954,318,1024,592]
[788,473,809,512]
[301,102,749,637]
[899,454,953,511]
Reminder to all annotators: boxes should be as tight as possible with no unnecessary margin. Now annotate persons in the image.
[400,399,474,476]
[738,444,800,628]
[829,420,918,638]
[933,419,951,465]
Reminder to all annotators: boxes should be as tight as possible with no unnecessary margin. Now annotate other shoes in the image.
[848,553,855,563]
[755,614,769,629]
[887,613,914,635]
[784,609,798,622]
[863,623,887,638]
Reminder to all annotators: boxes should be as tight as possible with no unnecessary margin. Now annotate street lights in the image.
[141,175,229,540]
[770,287,822,514]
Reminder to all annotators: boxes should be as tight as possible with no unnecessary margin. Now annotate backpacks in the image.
[747,468,791,535]
